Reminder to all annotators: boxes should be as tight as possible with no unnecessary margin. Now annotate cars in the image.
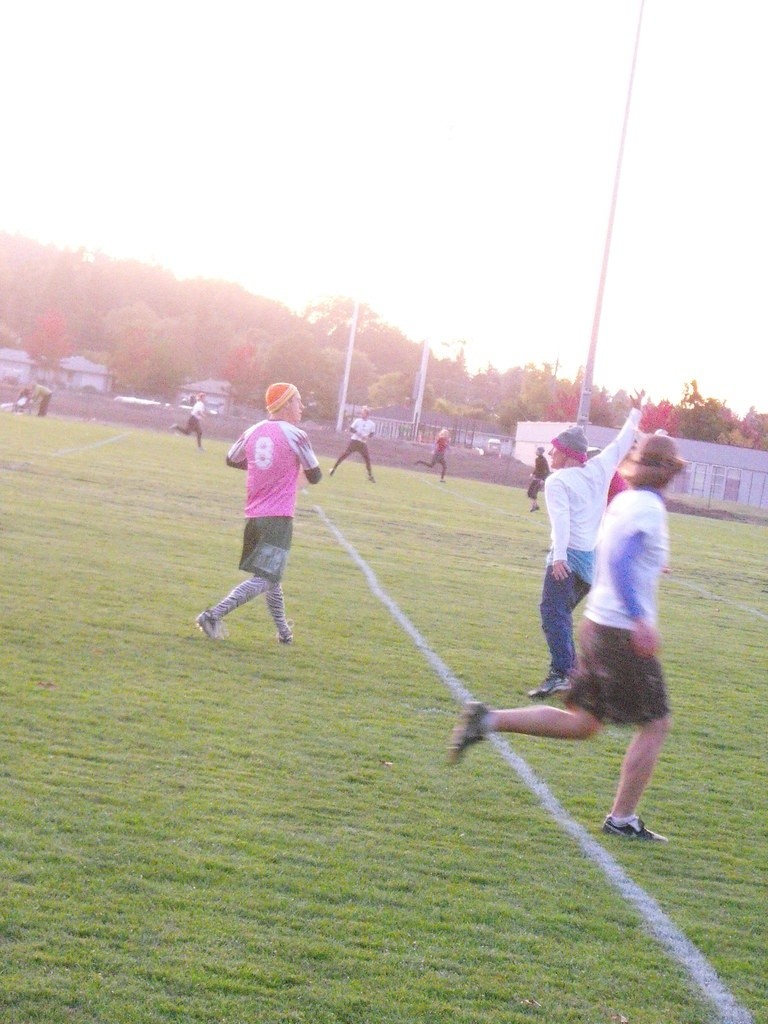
[487,438,502,457]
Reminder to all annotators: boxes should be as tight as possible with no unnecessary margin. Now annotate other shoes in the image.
[330,468,334,475]
[278,632,293,644]
[198,447,206,451]
[531,508,540,512]
[195,612,217,640]
[370,477,377,484]
[168,423,177,429]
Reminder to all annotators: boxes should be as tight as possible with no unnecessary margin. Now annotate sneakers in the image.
[528,673,572,700]
[448,701,489,766]
[601,818,671,845]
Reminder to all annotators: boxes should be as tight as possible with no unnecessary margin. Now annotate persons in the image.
[197,382,322,644]
[414,429,451,482]
[448,389,689,844]
[329,405,376,485]
[166,392,208,450]
[13,380,52,418]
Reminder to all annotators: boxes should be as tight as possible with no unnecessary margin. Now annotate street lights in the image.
[335,281,372,430]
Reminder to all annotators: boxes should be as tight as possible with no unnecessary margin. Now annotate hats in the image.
[265,381,297,414]
[551,427,588,463]
[616,433,694,487]
